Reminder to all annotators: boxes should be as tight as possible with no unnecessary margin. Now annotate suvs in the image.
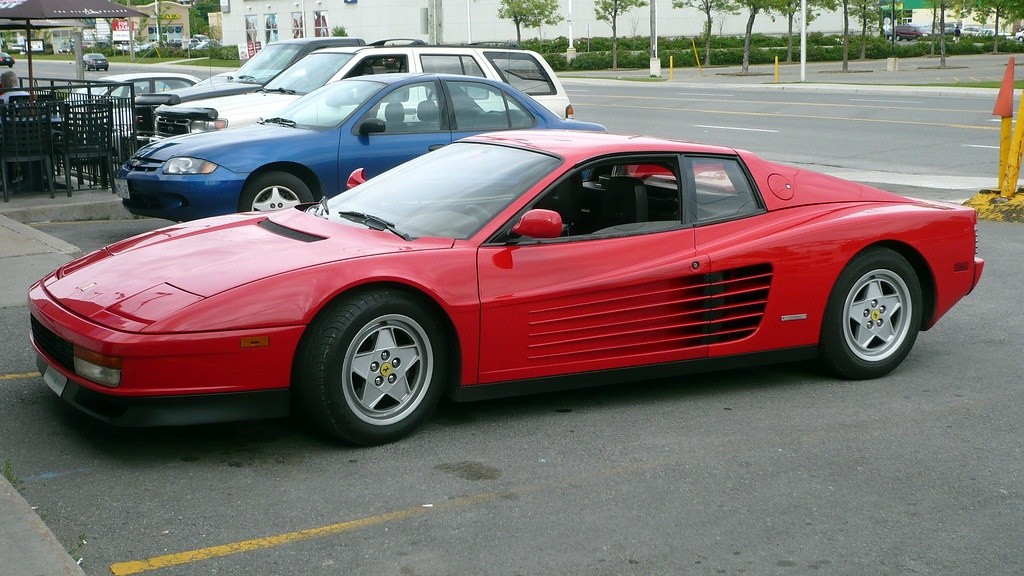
[149,36,575,137]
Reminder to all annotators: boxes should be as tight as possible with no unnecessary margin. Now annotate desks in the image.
[0,117,86,185]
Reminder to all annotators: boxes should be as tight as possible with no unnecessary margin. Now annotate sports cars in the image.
[23,131,986,449]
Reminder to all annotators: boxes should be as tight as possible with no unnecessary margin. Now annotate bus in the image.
[12,44,25,51]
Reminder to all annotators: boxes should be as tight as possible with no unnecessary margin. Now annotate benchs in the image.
[456,110,523,128]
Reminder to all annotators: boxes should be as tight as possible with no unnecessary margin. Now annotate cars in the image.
[114,41,151,53]
[190,34,222,49]
[0,52,15,68]
[64,71,202,130]
[118,72,610,228]
[882,19,1024,45]
[83,52,109,71]
[58,46,70,53]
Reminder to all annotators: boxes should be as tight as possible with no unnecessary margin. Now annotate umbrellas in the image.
[0,0,152,114]
[0,19,90,32]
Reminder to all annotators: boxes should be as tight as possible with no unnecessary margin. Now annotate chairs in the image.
[0,102,56,203]
[385,102,408,131]
[532,166,583,224]
[591,176,648,232]
[413,101,439,130]
[8,94,53,117]
[53,101,117,197]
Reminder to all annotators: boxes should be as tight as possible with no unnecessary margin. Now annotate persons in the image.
[0,70,37,186]
[955,25,960,44]
